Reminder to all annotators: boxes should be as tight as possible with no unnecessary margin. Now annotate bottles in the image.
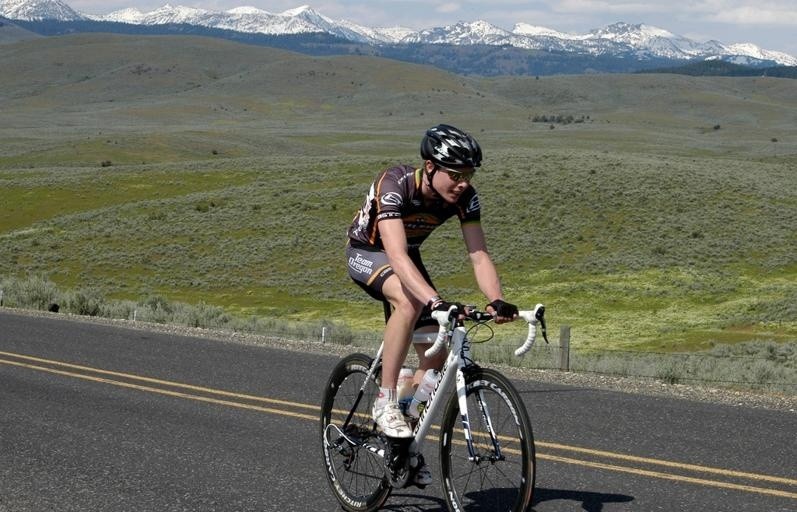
[396,366,413,410]
[407,368,442,420]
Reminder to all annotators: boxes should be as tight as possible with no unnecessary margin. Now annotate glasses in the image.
[443,170,475,181]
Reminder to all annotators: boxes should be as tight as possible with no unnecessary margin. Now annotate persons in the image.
[345,124,520,486]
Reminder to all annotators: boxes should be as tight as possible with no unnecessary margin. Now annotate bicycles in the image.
[319,271,549,512]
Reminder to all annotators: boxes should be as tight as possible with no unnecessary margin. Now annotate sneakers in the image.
[371,401,414,439]
[412,456,433,486]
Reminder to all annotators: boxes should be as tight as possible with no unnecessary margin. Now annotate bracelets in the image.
[426,295,441,310]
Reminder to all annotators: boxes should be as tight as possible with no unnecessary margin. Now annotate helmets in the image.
[422,125,483,172]
